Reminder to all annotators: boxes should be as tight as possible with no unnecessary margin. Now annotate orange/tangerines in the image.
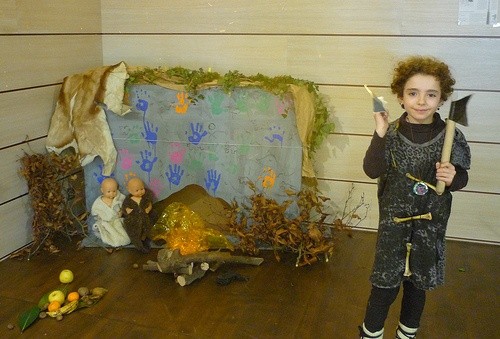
[48,301,60,312]
[68,292,80,302]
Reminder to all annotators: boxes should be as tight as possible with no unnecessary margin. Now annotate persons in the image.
[122,177,153,252]
[91,178,132,246]
[358,54,471,339]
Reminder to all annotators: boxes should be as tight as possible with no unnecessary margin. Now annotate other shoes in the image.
[396,321,419,339]
[359,322,385,339]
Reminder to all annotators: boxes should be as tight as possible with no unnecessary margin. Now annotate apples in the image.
[48,291,65,305]
[59,269,73,284]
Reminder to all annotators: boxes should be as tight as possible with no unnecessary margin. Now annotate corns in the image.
[56,301,80,315]
